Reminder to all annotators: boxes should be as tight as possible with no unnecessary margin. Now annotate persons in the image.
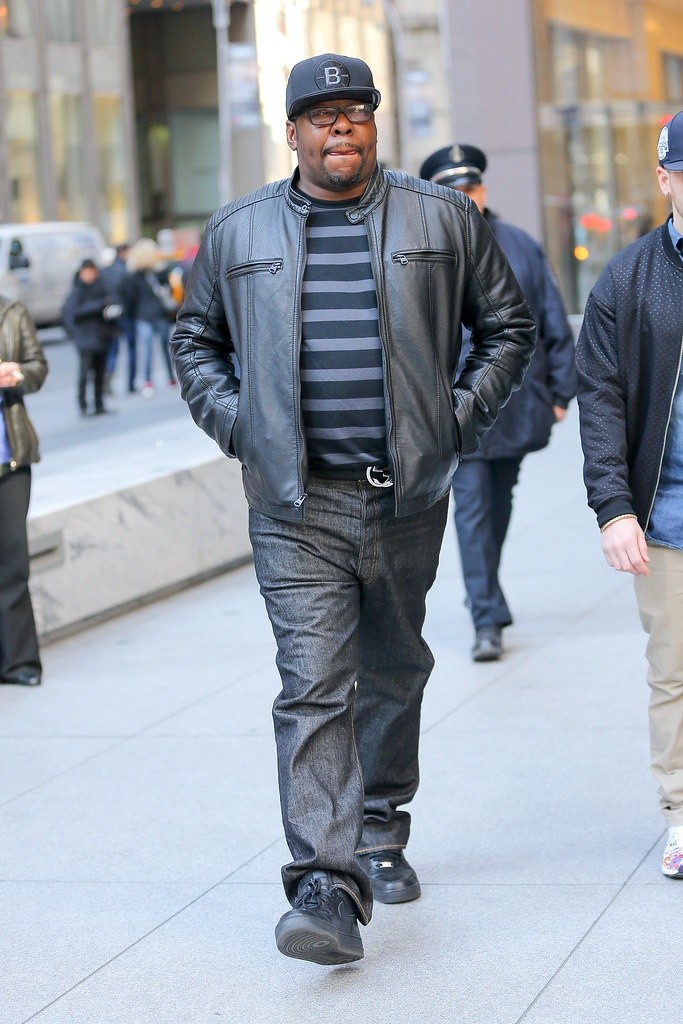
[418,142,574,660]
[64,226,205,416]
[0,295,49,686]
[169,52,538,966]
[575,112,683,879]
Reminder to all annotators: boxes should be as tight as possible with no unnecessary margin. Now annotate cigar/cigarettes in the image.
[11,370,25,381]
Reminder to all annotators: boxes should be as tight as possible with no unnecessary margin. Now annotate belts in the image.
[308,464,395,488]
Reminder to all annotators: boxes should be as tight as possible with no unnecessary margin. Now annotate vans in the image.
[0,222,120,325]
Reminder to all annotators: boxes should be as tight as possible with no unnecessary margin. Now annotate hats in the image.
[286,53,381,119]
[657,111,683,172]
[420,144,487,188]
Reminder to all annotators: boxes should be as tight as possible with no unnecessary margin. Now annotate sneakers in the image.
[354,847,420,904]
[275,871,363,966]
[661,826,683,879]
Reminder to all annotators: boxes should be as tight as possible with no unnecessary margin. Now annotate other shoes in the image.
[10,668,42,685]
[472,624,503,660]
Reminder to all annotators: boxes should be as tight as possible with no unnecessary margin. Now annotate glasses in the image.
[290,102,373,126]
